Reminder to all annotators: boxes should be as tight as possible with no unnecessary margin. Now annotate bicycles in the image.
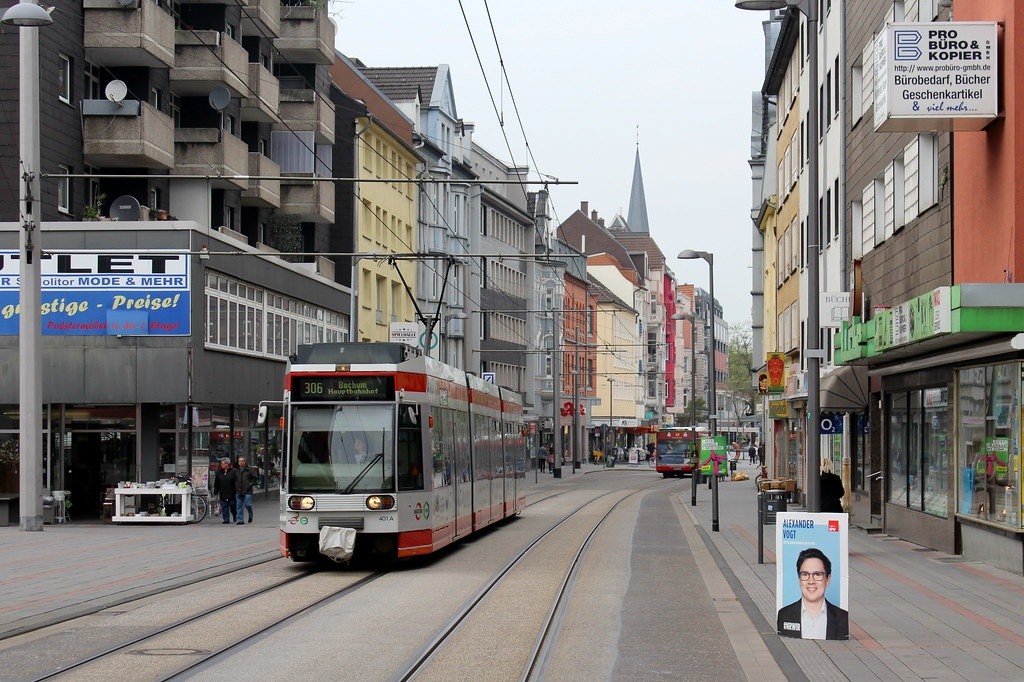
[173,473,207,523]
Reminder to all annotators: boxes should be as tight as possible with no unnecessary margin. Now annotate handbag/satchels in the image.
[214,495,222,516]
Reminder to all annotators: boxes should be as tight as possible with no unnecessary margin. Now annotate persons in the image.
[820,458,845,512]
[159,447,167,477]
[233,456,258,525]
[748,444,765,466]
[346,437,385,463]
[213,457,237,524]
[645,442,657,463]
[776,549,849,641]
[537,443,554,474]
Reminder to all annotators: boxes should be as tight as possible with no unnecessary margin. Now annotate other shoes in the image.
[222,521,231,524]
[233,514,237,522]
[237,522,245,525]
[248,517,253,523]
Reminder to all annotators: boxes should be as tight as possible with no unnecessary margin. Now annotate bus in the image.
[257,343,526,569]
[657,427,709,477]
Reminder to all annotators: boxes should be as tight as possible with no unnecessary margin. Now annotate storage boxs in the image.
[975,436,1018,478]
[762,477,796,491]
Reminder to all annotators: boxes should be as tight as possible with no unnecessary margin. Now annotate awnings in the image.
[817,363,871,416]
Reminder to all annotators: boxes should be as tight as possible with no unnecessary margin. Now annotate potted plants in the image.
[81,206,98,221]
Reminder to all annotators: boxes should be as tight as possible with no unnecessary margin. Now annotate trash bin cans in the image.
[730,460,736,470]
[766,489,787,524]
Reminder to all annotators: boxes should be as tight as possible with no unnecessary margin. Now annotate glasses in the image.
[799,572,825,581]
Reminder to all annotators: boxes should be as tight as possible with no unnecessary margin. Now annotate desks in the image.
[0,493,18,526]
[112,488,194,523]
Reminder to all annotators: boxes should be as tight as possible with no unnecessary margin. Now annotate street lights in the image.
[0,1,53,530]
[606,378,615,458]
[350,252,385,343]
[677,249,720,532]
[670,313,697,507]
[736,0,822,513]
[444,312,469,363]
[568,370,579,473]
